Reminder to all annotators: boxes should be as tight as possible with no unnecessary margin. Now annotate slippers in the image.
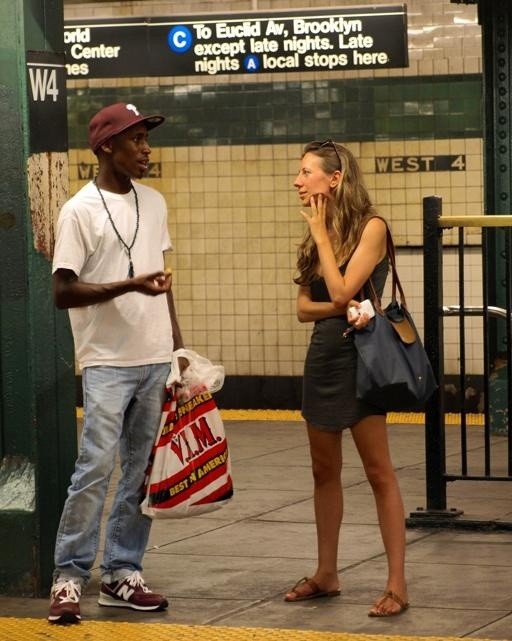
[284,573,344,601]
[366,590,413,620]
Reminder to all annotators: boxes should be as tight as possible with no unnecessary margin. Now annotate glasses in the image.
[321,137,342,171]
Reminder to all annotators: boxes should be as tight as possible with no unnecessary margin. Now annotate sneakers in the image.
[98,569,171,613]
[48,576,86,623]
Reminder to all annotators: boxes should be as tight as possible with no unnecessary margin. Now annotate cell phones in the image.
[349,299,375,323]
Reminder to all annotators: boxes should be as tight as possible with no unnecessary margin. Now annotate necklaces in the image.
[93,174,141,281]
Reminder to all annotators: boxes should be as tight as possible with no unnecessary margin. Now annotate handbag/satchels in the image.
[137,387,235,521]
[349,298,445,413]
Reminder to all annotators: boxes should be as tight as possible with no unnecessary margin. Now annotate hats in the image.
[87,97,167,153]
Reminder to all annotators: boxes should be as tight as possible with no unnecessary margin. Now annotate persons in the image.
[46,101,194,626]
[282,141,410,620]
